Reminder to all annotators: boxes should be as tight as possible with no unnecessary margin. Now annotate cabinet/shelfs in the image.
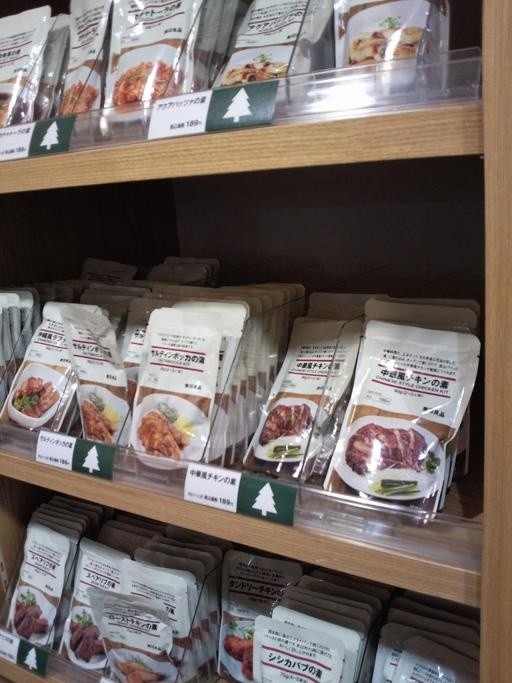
[0,2,511,683]
